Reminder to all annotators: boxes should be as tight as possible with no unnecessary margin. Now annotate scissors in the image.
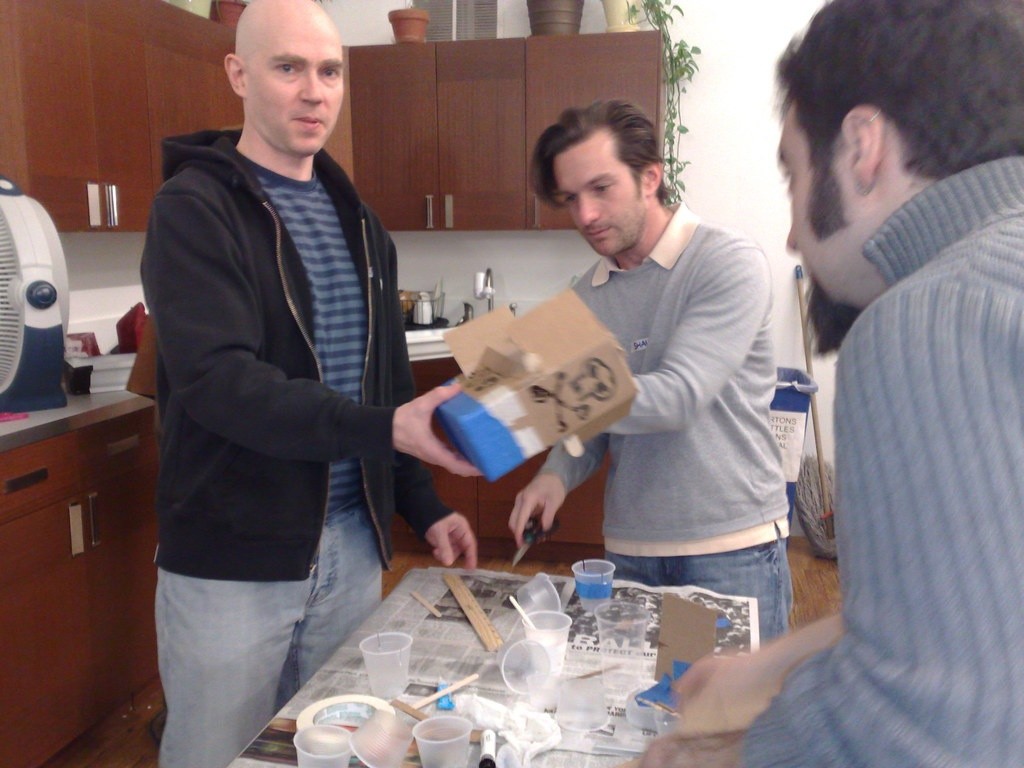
[513,518,560,567]
[0,412,28,423]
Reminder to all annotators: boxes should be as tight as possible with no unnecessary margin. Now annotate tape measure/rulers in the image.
[442,574,503,653]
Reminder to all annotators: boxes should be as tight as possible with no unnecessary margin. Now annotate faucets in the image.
[484,265,494,314]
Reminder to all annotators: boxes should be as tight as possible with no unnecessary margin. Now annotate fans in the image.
[1,178,71,409]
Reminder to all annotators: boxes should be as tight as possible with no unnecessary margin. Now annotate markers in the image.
[479,729,496,768]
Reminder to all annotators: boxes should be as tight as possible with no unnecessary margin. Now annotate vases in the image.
[388,10,428,43]
[526,0,585,34]
[211,0,246,28]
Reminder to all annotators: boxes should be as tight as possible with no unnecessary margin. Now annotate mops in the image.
[792,264,837,557]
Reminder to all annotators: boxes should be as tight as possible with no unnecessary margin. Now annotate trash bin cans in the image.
[768,367,818,551]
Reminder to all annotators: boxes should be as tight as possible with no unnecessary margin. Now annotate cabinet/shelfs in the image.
[2,354,628,766]
[2,0,670,234]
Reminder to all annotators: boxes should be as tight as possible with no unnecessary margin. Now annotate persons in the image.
[627,0,1024,768]
[139,0,486,768]
[508,100,792,645]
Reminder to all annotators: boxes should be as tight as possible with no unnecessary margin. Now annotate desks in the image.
[228,567,759,768]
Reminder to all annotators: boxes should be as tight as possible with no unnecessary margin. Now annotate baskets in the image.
[400,290,445,325]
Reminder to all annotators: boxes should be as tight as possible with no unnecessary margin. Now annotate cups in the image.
[571,559,616,611]
[398,290,433,325]
[517,572,561,614]
[350,710,414,768]
[555,678,611,732]
[359,632,413,699]
[412,715,473,768]
[522,610,572,674]
[594,601,651,692]
[293,726,352,768]
[497,639,550,696]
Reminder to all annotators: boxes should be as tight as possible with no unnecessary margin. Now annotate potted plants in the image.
[602,0,701,204]
